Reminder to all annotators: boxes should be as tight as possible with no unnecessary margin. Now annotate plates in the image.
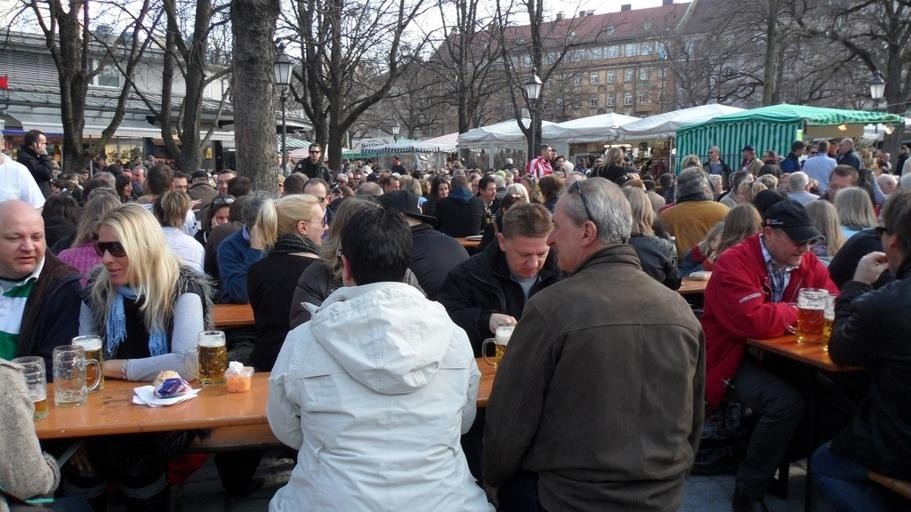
[465,235,482,241]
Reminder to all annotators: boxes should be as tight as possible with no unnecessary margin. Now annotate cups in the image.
[181,330,228,388]
[482,325,517,369]
[10,356,48,423]
[52,344,101,409]
[784,287,839,354]
[72,334,105,394]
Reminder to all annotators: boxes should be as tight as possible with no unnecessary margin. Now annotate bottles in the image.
[670,236,678,262]
[484,213,495,230]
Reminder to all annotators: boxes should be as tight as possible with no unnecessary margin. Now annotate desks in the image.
[36,236,860,440]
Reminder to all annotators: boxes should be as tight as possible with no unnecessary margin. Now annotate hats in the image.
[188,171,208,184]
[412,170,426,179]
[762,200,825,243]
[676,167,705,196]
[738,145,754,151]
[379,190,440,224]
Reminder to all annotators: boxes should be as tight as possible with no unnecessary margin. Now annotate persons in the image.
[2,130,911,512]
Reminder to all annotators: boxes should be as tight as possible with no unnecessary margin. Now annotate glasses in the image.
[568,181,593,222]
[310,151,320,154]
[307,216,328,227]
[212,197,234,208]
[94,242,127,258]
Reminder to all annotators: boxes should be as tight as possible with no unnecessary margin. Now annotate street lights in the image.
[391,119,399,142]
[521,67,543,160]
[272,41,295,181]
[868,69,884,148]
